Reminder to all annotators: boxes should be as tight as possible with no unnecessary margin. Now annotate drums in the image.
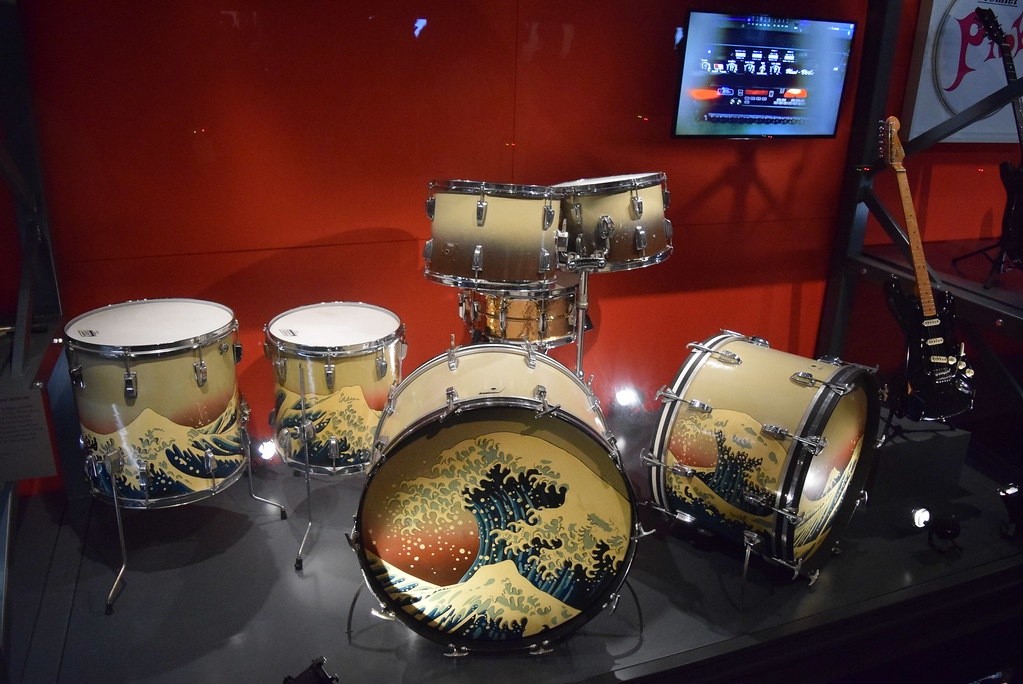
[653,330,886,570]
[62,171,670,654]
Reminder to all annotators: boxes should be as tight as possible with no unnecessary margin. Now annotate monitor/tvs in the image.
[671,8,857,139]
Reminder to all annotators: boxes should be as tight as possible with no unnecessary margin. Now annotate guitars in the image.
[978,3,1021,275]
[880,116,975,420]
